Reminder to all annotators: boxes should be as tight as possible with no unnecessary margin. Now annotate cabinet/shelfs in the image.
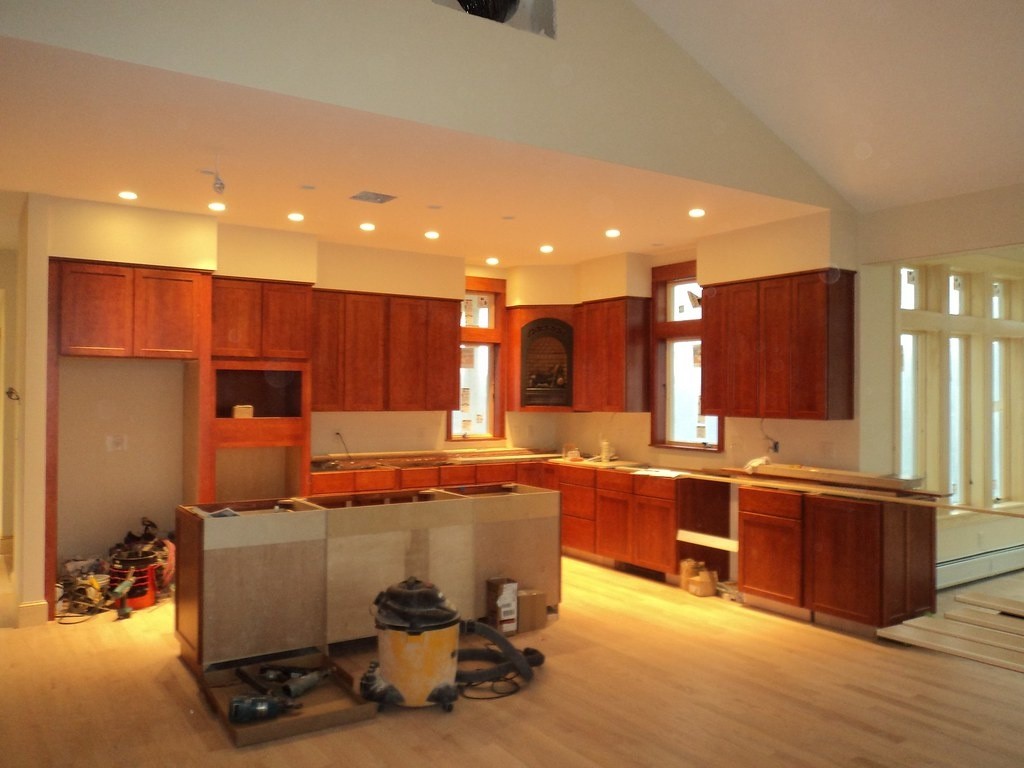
[315,454,941,625]
[567,298,655,418]
[47,257,464,413]
[701,267,860,429]
